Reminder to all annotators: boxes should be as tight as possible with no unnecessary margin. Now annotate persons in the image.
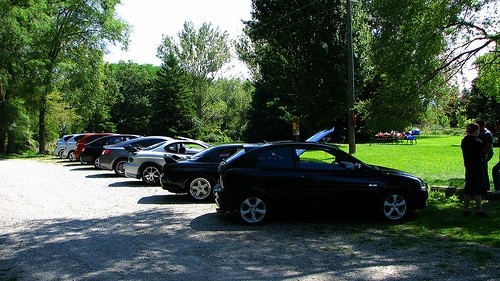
[462,118,500,220]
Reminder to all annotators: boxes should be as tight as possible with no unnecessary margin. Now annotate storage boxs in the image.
[412,129,420,135]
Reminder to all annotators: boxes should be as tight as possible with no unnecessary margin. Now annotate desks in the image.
[370,136,417,145]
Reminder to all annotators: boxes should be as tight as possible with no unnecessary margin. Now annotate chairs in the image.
[179,147,186,154]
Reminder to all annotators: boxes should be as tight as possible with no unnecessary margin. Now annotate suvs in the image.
[213,140,429,227]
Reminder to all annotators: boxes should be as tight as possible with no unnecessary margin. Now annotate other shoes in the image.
[463,211,471,217]
[474,211,489,218]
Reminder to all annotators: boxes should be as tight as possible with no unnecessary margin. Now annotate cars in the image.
[159,142,264,203]
[124,139,209,186]
[53,133,113,162]
[80,134,144,171]
[99,136,174,177]
